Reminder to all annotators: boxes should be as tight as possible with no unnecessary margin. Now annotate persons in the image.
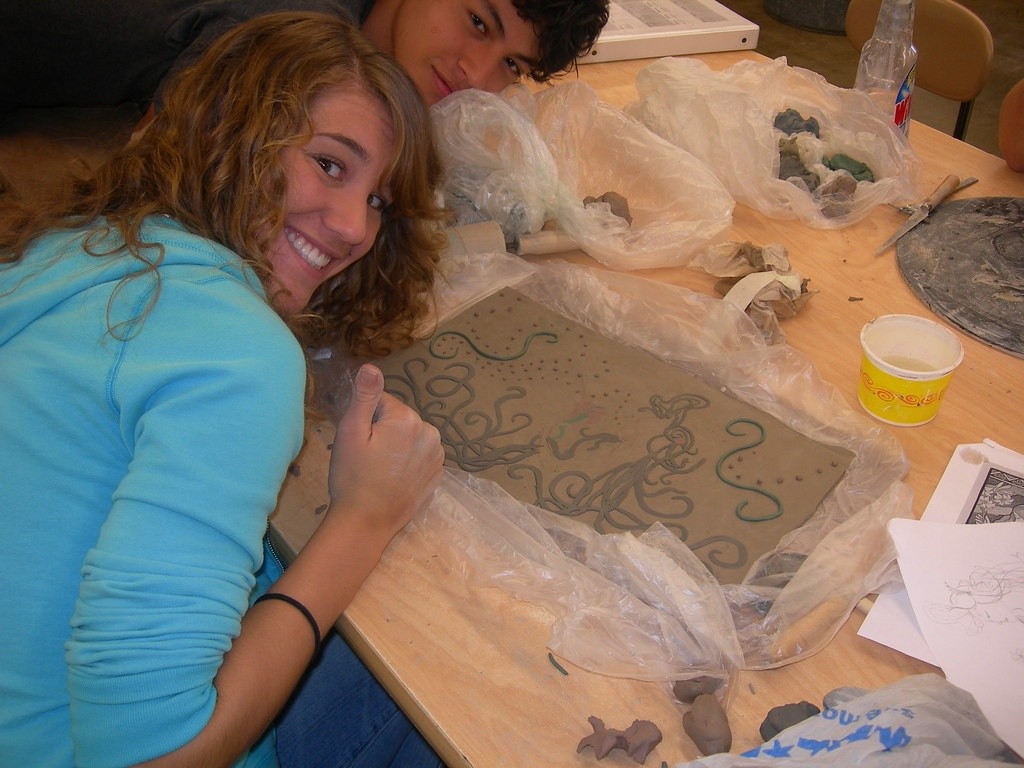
[0,11,460,768]
[0,1,612,143]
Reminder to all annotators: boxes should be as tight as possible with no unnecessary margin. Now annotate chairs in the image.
[843,1,994,140]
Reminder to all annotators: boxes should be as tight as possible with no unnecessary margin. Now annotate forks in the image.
[887,176,979,216]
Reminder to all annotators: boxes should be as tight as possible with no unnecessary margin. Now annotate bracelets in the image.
[252,593,345,663]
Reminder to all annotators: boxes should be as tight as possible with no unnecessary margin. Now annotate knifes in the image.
[872,174,961,253]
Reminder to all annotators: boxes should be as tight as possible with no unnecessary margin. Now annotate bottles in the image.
[854,0,920,141]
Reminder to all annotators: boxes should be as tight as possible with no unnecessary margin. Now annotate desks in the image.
[1,49,1024,768]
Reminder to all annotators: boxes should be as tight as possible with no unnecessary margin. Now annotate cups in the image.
[857,313,966,428]
